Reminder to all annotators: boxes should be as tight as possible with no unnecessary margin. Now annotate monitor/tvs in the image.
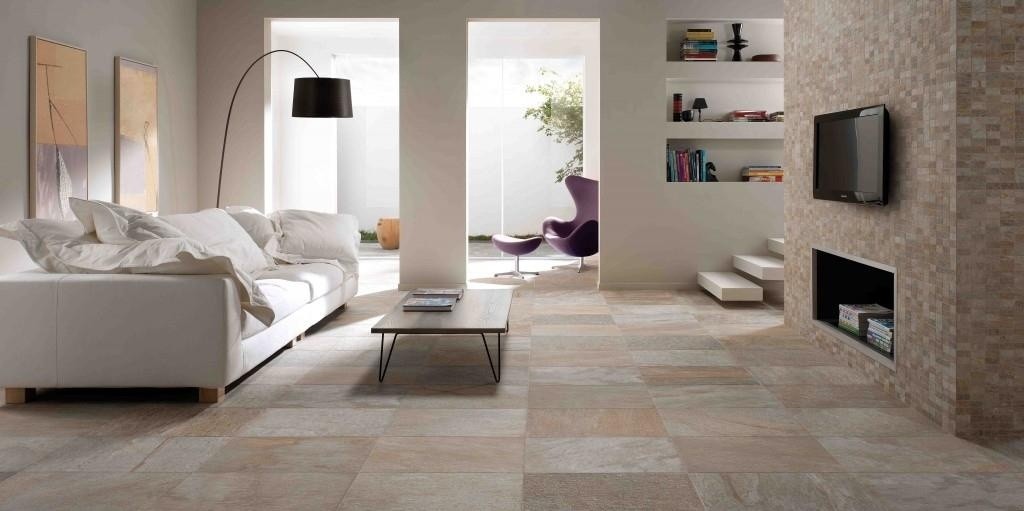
[812,103,889,208]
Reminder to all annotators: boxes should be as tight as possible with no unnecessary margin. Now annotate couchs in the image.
[0,214,361,404]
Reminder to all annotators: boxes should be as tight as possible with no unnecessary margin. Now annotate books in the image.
[410,287,464,300]
[666,143,785,183]
[837,303,894,354]
[727,109,785,122]
[676,27,718,62]
[402,297,457,312]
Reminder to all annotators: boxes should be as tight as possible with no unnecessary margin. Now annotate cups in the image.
[681,110,694,122]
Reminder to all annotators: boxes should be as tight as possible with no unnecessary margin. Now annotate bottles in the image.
[672,92,684,121]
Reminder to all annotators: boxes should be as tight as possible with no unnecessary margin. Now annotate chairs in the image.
[542,175,599,273]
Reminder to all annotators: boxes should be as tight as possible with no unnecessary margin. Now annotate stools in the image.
[492,234,543,280]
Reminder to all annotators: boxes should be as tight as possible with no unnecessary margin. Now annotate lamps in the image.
[216,50,353,209]
[692,98,708,122]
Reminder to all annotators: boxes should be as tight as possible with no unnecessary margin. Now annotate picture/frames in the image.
[114,55,160,218]
[28,34,91,221]
